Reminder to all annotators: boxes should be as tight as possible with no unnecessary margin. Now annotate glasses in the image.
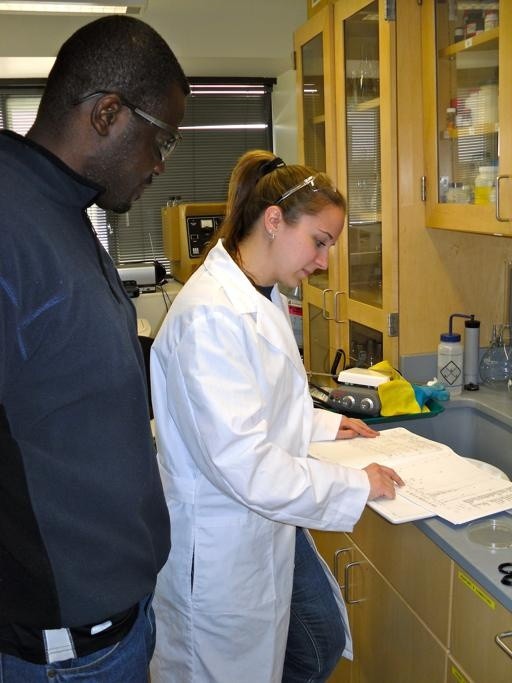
[77,90,184,161]
[275,170,335,209]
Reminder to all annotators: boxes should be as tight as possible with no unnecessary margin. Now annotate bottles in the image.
[437,313,477,397]
[439,8,499,203]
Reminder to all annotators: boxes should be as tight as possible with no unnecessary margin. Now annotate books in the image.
[306,423,512,525]
[363,457,510,524]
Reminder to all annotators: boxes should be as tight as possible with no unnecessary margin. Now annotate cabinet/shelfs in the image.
[290,1,395,373]
[308,504,512,683]
[421,2,512,238]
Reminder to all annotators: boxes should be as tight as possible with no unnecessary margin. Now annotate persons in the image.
[3,12,195,683]
[155,150,408,683]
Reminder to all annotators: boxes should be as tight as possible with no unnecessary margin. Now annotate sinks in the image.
[362,398,512,516]
